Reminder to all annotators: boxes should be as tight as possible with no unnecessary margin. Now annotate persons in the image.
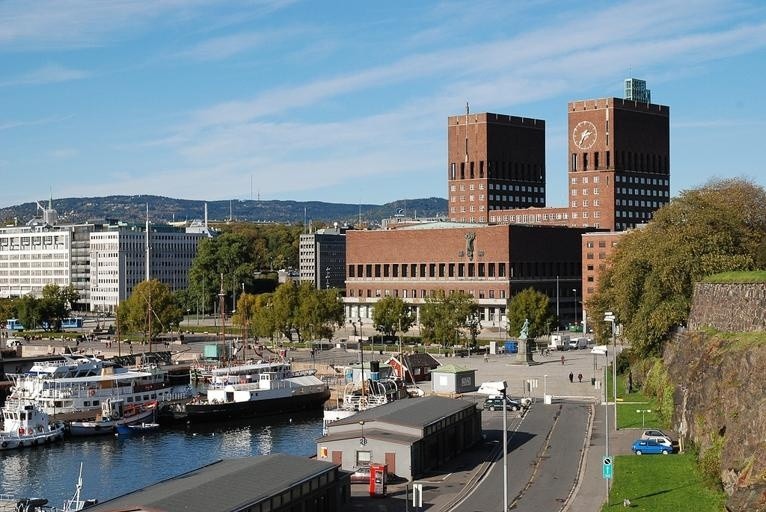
[81,334,95,343]
[578,374,583,382]
[569,371,573,383]
[5,332,8,338]
[310,346,320,358]
[483,352,488,363]
[23,332,42,342]
[129,344,133,355]
[2,331,4,338]
[521,319,530,336]
[49,335,55,341]
[105,341,112,349]
[560,354,564,364]
[75,338,79,346]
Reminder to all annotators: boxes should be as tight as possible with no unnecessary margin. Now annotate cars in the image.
[483,395,520,411]
[631,429,673,455]
[350,466,382,484]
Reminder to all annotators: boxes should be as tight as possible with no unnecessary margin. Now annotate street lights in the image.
[572,288,577,321]
[604,312,617,430]
[589,345,610,506]
[476,380,508,512]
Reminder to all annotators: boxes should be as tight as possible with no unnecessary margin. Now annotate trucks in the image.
[551,335,587,350]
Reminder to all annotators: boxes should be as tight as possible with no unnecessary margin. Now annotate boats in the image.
[0,281,332,450]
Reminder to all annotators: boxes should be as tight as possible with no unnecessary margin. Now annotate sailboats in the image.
[322,312,424,435]
